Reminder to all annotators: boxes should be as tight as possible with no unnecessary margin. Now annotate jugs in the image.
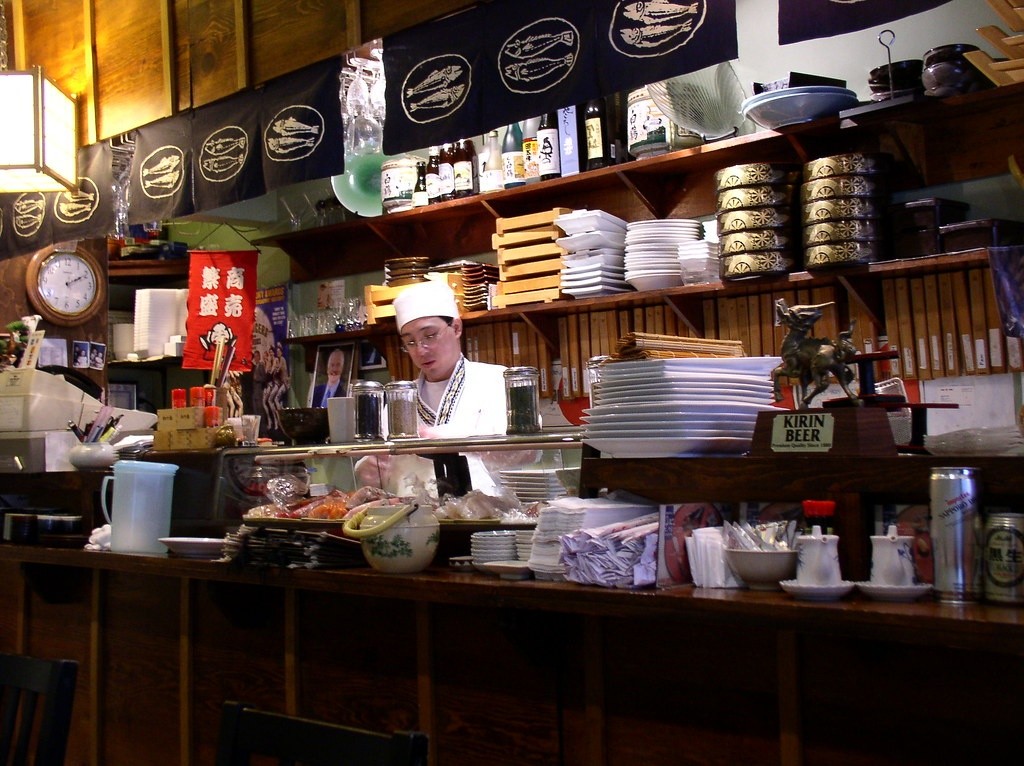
[101,459,179,554]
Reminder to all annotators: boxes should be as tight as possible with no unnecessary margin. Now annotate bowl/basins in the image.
[724,549,797,590]
[158,537,228,558]
[69,442,114,470]
[278,408,329,446]
[869,44,982,102]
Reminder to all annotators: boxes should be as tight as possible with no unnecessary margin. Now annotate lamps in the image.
[0,65,79,193]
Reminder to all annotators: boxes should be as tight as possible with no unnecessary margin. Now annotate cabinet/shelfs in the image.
[248,72,1024,376]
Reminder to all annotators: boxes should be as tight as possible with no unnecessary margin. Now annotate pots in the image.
[627,81,705,162]
[380,155,425,214]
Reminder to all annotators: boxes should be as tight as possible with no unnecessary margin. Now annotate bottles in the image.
[503,366,541,434]
[537,110,561,181]
[523,118,541,183]
[584,97,615,171]
[426,146,439,204]
[501,123,526,189]
[384,380,419,438]
[439,142,454,202]
[454,139,473,199]
[477,130,502,194]
[413,161,429,206]
[171,385,224,429]
[351,380,384,439]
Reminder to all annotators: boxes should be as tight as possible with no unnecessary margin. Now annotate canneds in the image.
[928,466,984,605]
[983,512,1024,604]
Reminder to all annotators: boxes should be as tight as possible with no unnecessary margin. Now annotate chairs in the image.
[0,648,81,766]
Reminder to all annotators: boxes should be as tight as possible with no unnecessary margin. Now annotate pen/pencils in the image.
[68,404,125,443]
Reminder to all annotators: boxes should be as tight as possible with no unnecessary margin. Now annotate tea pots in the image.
[796,525,842,584]
[869,525,915,586]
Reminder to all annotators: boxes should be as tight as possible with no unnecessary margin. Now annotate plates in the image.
[580,357,790,458]
[499,467,579,502]
[462,263,499,310]
[383,256,430,287]
[554,211,719,299]
[470,530,534,580]
[856,580,932,599]
[778,579,854,599]
[740,86,859,127]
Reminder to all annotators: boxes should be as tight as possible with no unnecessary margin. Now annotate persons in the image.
[90,349,104,369]
[225,345,289,433]
[74,346,88,366]
[312,349,346,408]
[355,282,544,507]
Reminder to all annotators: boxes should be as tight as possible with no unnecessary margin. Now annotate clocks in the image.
[25,242,106,327]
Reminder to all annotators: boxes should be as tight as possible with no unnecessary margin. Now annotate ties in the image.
[322,390,330,408]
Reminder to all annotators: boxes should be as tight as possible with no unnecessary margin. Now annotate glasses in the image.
[400,321,452,353]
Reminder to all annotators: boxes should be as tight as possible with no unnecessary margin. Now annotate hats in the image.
[392,281,460,332]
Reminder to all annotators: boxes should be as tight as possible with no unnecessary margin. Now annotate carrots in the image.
[309,493,351,519]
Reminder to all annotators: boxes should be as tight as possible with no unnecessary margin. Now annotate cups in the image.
[327,397,356,443]
